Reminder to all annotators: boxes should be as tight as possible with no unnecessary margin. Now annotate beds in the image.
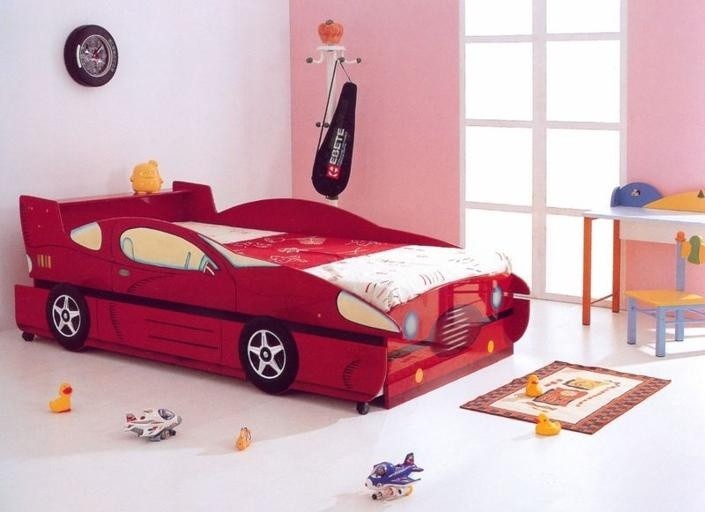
[13,157,535,420]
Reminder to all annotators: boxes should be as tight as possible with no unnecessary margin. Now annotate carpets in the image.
[458,357,675,437]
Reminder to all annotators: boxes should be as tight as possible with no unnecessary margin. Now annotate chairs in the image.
[623,233,705,358]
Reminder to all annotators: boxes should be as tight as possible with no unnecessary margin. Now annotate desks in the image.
[577,179,705,329]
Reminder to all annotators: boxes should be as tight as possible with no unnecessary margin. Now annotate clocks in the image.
[65,23,120,87]
